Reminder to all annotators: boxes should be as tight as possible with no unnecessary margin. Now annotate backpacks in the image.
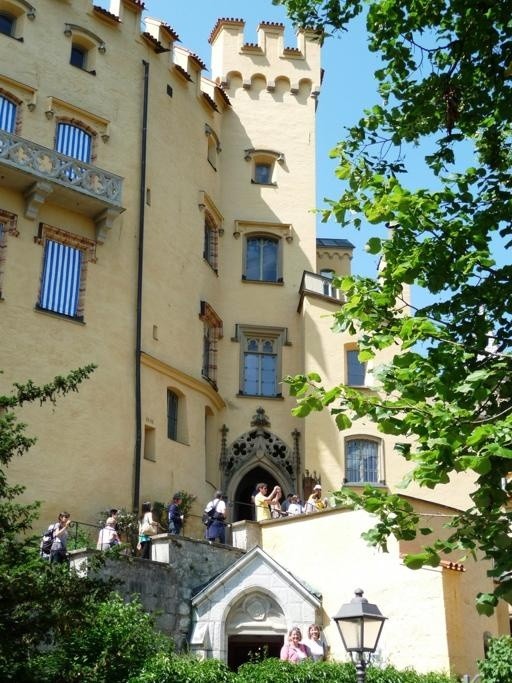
[41,523,62,553]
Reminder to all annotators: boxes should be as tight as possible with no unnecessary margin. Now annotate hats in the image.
[314,484,323,490]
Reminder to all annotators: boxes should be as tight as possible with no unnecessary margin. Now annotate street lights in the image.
[331,587,387,682]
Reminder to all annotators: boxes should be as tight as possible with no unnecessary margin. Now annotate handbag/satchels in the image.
[201,509,215,527]
[142,523,159,536]
[111,540,125,553]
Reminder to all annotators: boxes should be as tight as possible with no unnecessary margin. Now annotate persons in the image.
[279,626,307,667]
[167,493,184,534]
[137,500,161,559]
[249,481,330,522]
[96,516,123,552]
[110,508,121,539]
[284,623,328,662]
[48,510,72,565]
[203,490,228,545]
